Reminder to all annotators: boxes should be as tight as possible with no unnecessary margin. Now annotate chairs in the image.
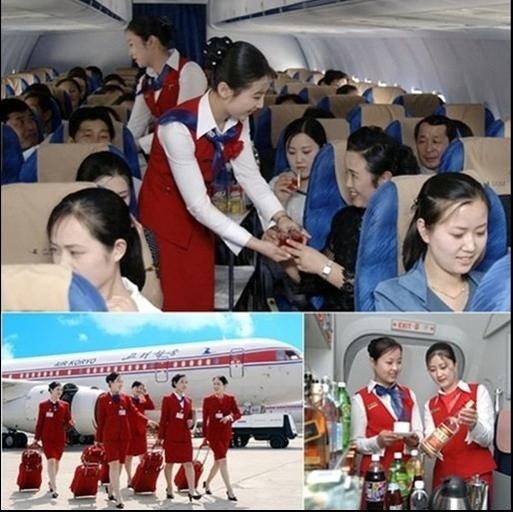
[241,67,510,312]
[0,62,163,312]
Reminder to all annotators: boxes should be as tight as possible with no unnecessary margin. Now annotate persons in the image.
[158,375,202,502]
[125,381,156,488]
[202,375,238,501]
[0,15,490,314]
[34,381,71,497]
[94,371,159,508]
[424,342,495,510]
[351,337,425,510]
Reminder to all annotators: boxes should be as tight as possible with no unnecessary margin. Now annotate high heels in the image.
[188,493,202,501]
[166,489,174,499]
[227,492,237,501]
[203,482,211,494]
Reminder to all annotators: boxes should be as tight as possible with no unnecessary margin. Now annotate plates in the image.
[393,432,413,438]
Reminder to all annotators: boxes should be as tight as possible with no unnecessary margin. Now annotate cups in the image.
[393,421,410,432]
[278,225,303,247]
[284,167,301,192]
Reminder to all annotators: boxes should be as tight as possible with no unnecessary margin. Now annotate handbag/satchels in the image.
[22,451,41,465]
[81,445,106,467]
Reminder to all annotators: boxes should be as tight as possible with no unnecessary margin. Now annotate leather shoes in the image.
[48,482,58,498]
[109,496,124,508]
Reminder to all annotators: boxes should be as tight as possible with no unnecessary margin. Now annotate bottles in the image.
[419,400,475,455]
[366,449,428,511]
[304,370,353,470]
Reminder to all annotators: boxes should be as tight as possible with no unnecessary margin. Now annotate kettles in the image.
[432,475,473,511]
[465,471,492,511]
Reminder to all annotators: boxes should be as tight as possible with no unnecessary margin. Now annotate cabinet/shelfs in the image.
[304,312,335,380]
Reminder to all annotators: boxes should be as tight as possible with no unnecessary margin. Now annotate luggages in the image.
[71,444,109,500]
[17,443,42,492]
[175,444,211,492]
[131,443,166,494]
